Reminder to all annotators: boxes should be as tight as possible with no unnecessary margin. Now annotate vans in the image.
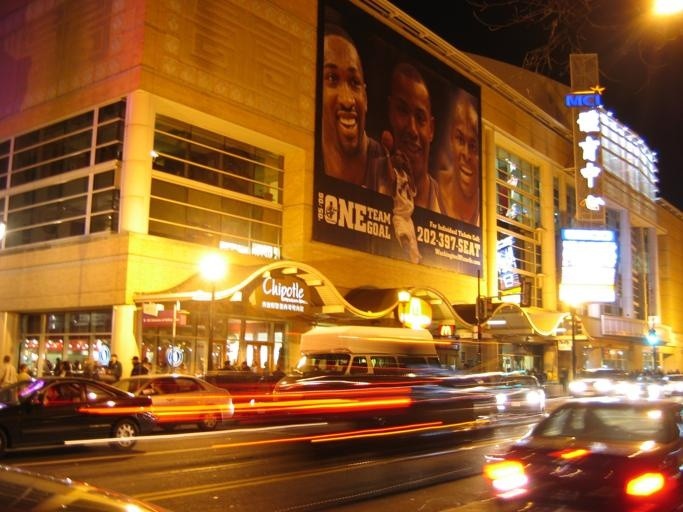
[274,322,441,393]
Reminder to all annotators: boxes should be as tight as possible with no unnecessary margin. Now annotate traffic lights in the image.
[647,328,657,346]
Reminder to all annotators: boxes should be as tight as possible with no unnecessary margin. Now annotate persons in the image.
[320,26,391,188]
[379,138,424,265]
[224,360,285,377]
[437,89,480,228]
[365,62,450,217]
[0,353,151,393]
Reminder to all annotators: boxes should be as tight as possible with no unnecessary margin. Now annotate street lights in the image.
[199,249,229,367]
[559,282,583,375]
[396,290,410,327]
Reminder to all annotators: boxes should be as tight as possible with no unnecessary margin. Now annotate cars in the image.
[574,367,682,395]
[481,395,683,512]
[0,373,152,450]
[496,371,545,414]
[110,372,234,431]
